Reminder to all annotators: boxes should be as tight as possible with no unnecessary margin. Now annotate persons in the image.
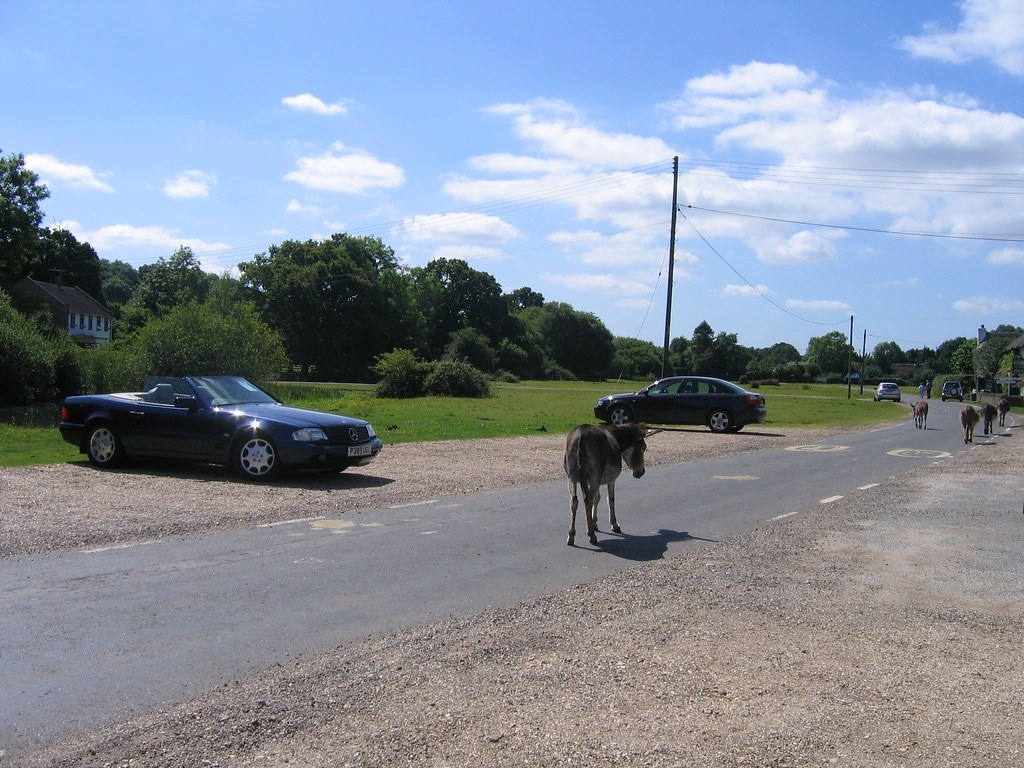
[926,380,932,399]
[918,382,925,399]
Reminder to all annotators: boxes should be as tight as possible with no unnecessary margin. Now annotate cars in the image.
[873,383,901,402]
[593,375,768,434]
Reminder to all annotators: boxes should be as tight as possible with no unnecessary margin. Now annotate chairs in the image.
[193,386,209,401]
[154,383,174,405]
[684,385,692,393]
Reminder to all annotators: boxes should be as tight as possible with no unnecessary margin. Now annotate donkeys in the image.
[959,397,1011,445]
[562,421,648,547]
[910,401,929,430]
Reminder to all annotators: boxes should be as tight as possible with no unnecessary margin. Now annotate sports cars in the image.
[61,375,383,484]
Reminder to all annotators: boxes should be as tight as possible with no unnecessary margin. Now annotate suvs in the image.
[940,380,964,402]
